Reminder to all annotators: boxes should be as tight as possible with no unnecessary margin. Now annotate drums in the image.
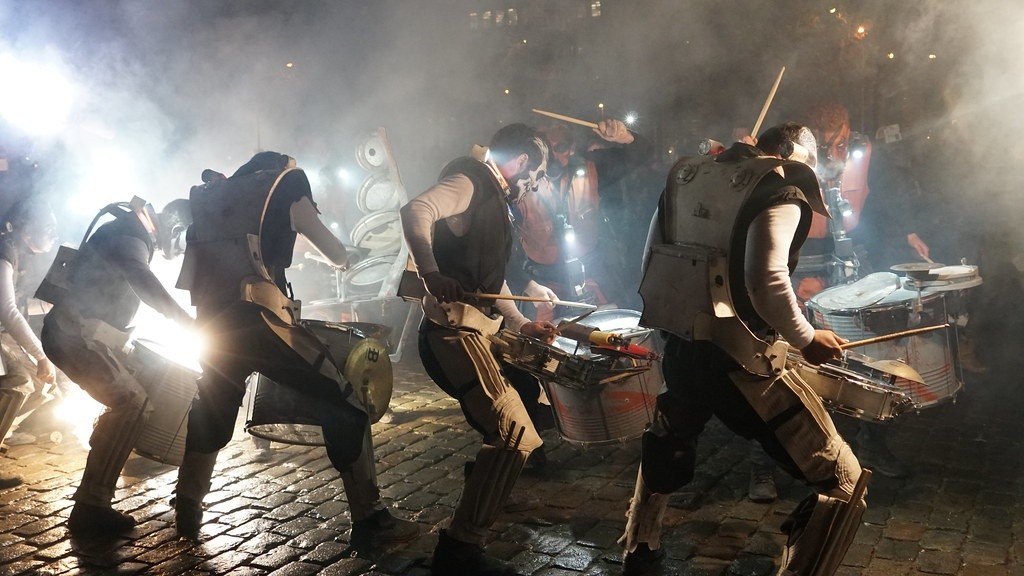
[928,264,983,300]
[130,336,203,467]
[493,330,593,392]
[296,128,422,361]
[548,302,619,328]
[808,275,964,411]
[244,319,366,446]
[538,308,664,447]
[787,353,905,425]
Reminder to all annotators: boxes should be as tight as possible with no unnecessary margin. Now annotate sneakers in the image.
[621,541,666,576]
[350,507,419,549]
[68,505,135,532]
[175,500,202,539]
[431,528,518,576]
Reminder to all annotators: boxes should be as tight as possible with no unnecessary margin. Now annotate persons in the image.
[398,121,563,576]
[617,119,871,575]
[0,206,59,486]
[505,116,650,471]
[170,152,421,550]
[736,104,931,502]
[42,199,193,536]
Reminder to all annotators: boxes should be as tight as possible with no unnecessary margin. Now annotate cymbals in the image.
[338,321,394,340]
[862,357,930,387]
[890,262,947,278]
[846,354,876,365]
[342,338,394,425]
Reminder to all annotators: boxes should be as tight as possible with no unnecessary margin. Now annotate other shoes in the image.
[0,474,23,489]
